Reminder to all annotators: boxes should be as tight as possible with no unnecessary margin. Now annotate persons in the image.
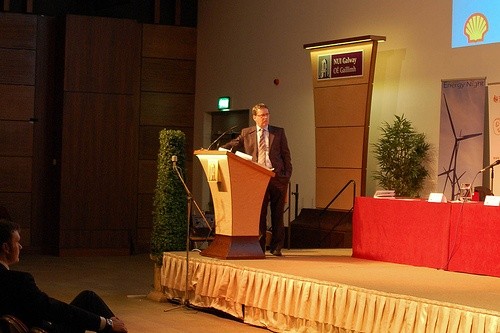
[231,103,293,256]
[0,219,127,333]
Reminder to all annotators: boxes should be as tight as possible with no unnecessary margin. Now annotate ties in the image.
[259,128,266,167]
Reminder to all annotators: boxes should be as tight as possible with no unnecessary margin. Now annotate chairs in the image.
[0,314,49,333]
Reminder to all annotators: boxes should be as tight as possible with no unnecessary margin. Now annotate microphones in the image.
[172,154,178,171]
[479,160,500,174]
[229,129,257,152]
[208,125,239,149]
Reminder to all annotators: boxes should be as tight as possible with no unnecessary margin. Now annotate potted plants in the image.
[149,129,186,290]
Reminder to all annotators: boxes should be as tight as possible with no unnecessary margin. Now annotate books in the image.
[374,190,396,199]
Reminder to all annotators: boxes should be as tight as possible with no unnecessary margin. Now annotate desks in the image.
[352,196,500,276]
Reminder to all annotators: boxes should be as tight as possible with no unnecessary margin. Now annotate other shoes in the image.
[270,249,281,256]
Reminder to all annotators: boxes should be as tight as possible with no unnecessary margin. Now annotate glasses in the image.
[257,113,270,117]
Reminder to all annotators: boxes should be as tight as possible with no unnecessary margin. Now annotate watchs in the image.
[103,318,113,332]
